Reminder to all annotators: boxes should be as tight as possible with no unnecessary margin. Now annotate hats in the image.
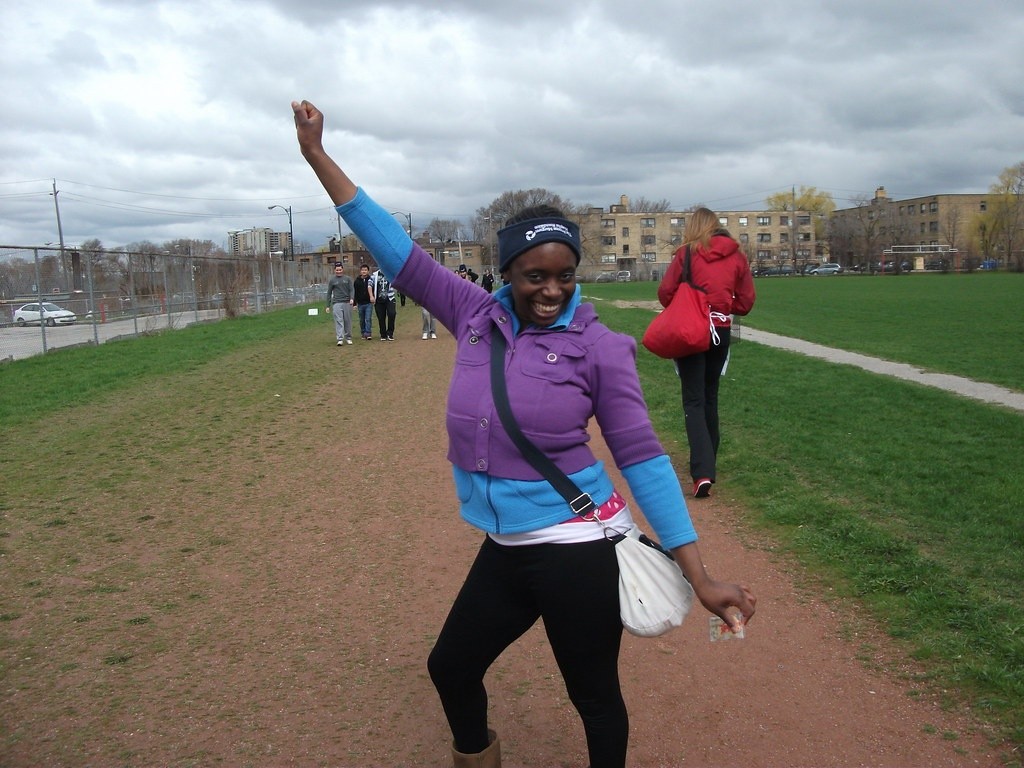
[459,264,466,273]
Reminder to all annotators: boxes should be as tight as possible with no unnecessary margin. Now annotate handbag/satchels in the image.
[604,524,693,638]
[642,245,712,357]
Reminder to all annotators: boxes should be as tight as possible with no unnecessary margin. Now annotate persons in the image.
[353,264,375,339]
[367,269,397,340]
[657,207,756,496]
[421,307,437,339]
[292,100,758,768]
[454,264,479,283]
[481,269,494,294]
[325,262,354,345]
[501,275,510,286]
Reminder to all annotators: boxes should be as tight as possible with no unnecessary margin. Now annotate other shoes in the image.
[361,332,366,340]
[337,340,343,346]
[430,333,437,338]
[381,336,387,341]
[366,332,372,339]
[422,332,428,339]
[347,339,353,345]
[387,334,394,341]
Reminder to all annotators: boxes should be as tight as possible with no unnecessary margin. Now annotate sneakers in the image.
[694,476,712,497]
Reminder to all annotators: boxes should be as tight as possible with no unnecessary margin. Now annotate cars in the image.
[617,270,631,283]
[804,265,821,274]
[752,266,770,275]
[211,282,327,304]
[761,265,799,276]
[925,258,948,269]
[810,263,845,275]
[850,260,913,272]
[595,271,617,283]
[12,302,78,327]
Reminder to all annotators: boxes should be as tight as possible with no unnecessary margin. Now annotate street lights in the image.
[174,244,198,322]
[483,209,494,281]
[268,204,295,264]
[391,211,412,240]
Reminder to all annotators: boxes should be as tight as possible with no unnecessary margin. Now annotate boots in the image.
[451,728,501,768]
